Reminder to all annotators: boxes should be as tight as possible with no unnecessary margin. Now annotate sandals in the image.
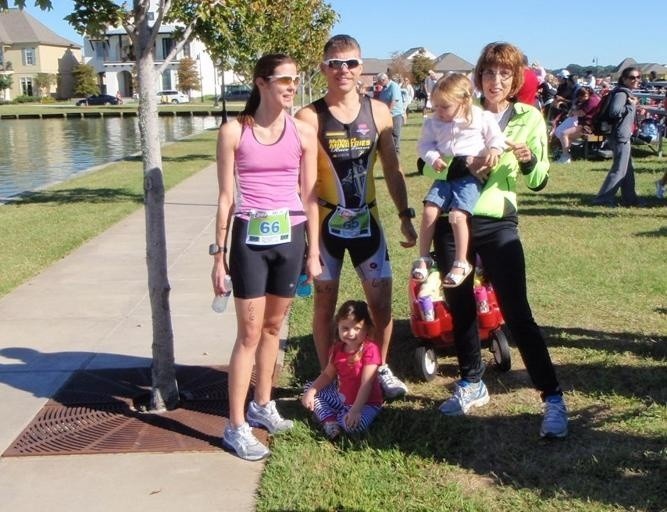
[442,260,473,288]
[412,256,434,282]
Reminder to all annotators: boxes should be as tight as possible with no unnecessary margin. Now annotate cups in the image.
[296,274,312,297]
[417,295,435,321]
[474,286,489,313]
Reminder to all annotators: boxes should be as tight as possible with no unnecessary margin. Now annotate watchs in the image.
[209,244,227,255]
[399,208,416,219]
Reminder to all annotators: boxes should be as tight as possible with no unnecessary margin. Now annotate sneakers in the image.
[324,421,339,438]
[438,379,490,416]
[540,395,568,438]
[377,363,407,398]
[222,400,293,461]
[656,181,664,198]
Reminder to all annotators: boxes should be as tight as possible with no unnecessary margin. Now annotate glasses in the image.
[627,76,640,79]
[322,58,363,68]
[266,75,298,85]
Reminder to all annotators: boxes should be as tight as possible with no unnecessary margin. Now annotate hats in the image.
[557,70,570,78]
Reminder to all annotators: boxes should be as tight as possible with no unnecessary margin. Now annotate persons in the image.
[116,91,121,105]
[432,41,569,439]
[211,53,323,462]
[411,71,505,289]
[293,34,417,400]
[297,300,383,440]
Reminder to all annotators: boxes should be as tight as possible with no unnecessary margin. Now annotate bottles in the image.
[211,276,233,313]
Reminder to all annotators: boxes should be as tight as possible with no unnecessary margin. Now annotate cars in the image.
[217,89,252,101]
[75,94,124,106]
[156,90,189,104]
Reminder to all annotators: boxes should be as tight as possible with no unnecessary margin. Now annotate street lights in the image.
[592,56,599,77]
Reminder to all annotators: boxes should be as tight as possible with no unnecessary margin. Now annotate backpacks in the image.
[592,89,629,135]
